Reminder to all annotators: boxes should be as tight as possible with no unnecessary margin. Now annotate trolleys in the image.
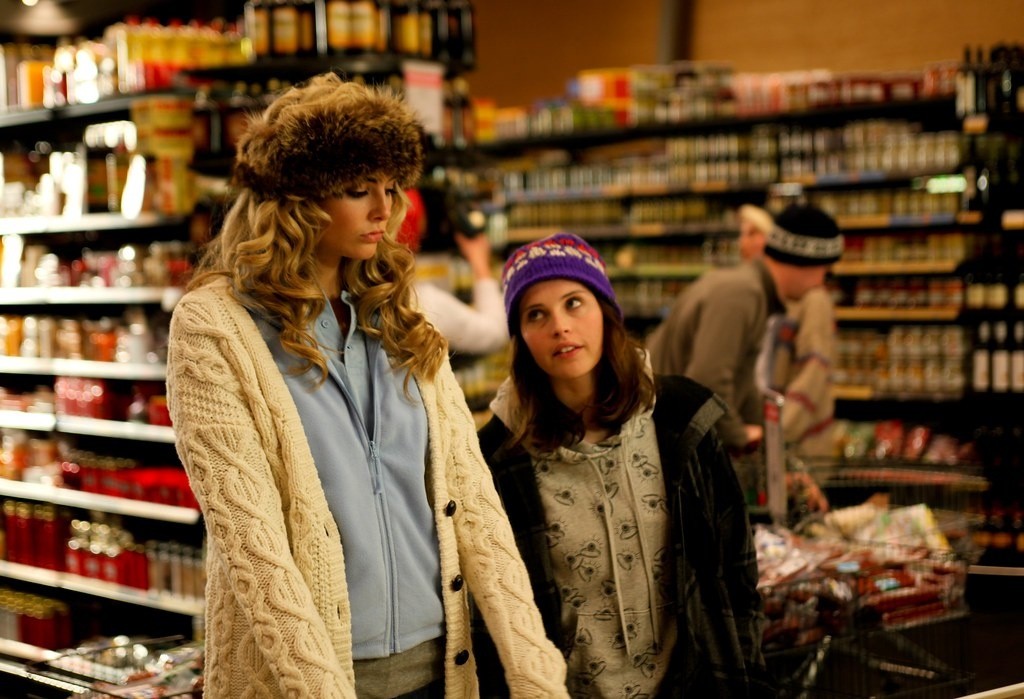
[744,402,985,698]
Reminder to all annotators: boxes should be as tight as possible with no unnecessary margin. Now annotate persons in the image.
[469,229,768,699]
[381,189,509,363]
[732,202,840,515]
[164,73,574,699]
[642,204,842,518]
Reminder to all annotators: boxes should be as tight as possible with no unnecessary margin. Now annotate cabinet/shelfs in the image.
[959,107,1024,576]
[479,70,982,563]
[0,0,479,699]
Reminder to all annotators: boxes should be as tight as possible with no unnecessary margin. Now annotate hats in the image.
[393,187,429,249]
[763,202,846,267]
[737,194,792,234]
[501,230,620,335]
